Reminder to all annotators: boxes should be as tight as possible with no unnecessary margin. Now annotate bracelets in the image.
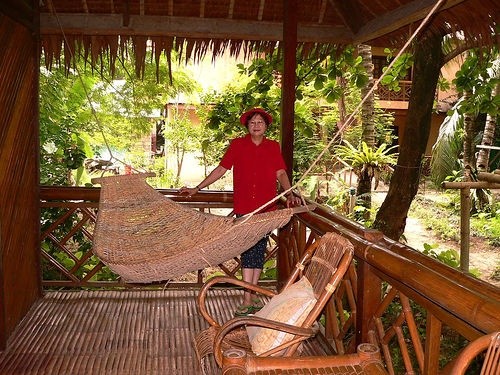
[197,186,201,191]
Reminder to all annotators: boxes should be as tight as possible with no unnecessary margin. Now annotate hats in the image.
[240,108,272,125]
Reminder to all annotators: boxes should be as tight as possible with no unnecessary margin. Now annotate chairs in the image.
[190,232,355,375]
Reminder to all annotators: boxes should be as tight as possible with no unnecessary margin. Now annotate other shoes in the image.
[235,305,252,317]
[251,297,263,310]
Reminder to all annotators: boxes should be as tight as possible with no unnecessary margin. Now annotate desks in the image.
[221,342,390,375]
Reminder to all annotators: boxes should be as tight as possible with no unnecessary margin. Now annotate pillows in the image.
[247,275,317,356]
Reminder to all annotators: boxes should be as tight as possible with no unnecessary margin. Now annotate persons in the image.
[179,108,301,317]
